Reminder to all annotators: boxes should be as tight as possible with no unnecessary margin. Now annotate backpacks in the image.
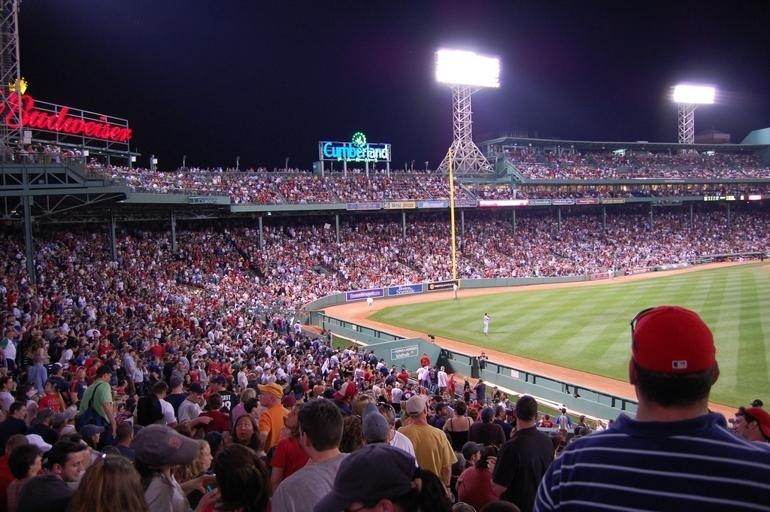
[73,382,113,443]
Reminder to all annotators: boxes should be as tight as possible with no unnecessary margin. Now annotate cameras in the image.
[204,484,213,492]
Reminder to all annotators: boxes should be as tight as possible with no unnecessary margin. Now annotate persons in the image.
[1,141,769,512]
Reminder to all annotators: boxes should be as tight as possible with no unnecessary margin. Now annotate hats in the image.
[211,376,226,384]
[26,433,54,451]
[81,423,105,437]
[435,402,451,409]
[255,381,285,399]
[51,362,68,371]
[629,304,717,375]
[313,446,416,510]
[190,382,206,393]
[129,425,201,471]
[736,399,769,437]
[361,401,389,444]
[461,440,485,457]
[405,392,434,417]
